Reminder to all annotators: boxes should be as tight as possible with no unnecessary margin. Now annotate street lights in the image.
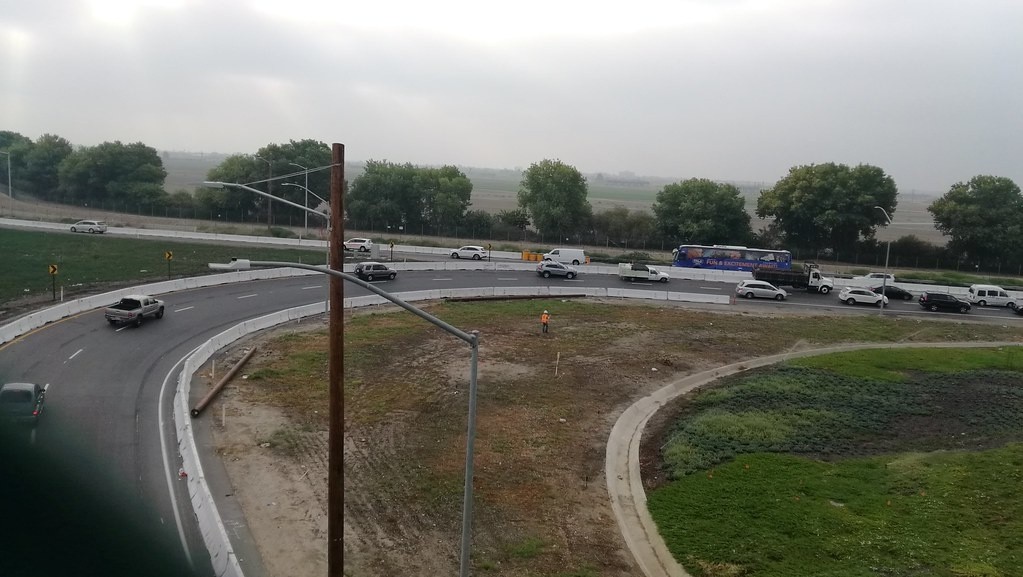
[289,163,307,237]
[873,206,893,317]
[207,258,478,577]
[203,181,330,317]
[257,157,271,231]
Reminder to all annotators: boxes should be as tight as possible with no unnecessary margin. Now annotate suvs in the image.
[343,238,373,252]
[354,262,397,282]
[919,292,972,314]
[536,261,577,280]
[450,246,487,260]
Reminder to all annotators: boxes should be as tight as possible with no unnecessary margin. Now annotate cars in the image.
[735,279,787,301]
[70,219,107,233]
[0,383,46,426]
[1013,297,1023,315]
[870,285,913,301]
[838,287,889,307]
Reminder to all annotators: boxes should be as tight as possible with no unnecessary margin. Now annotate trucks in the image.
[618,263,670,283]
[543,249,585,265]
[752,263,833,294]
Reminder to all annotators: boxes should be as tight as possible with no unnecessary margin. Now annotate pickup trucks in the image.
[103,295,164,328]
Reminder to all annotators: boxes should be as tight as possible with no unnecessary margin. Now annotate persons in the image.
[541,309,550,334]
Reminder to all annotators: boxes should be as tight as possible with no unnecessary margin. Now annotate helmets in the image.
[544,310,548,313]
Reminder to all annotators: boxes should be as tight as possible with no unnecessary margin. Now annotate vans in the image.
[967,284,1017,308]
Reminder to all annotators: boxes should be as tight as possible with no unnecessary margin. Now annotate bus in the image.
[672,245,792,272]
